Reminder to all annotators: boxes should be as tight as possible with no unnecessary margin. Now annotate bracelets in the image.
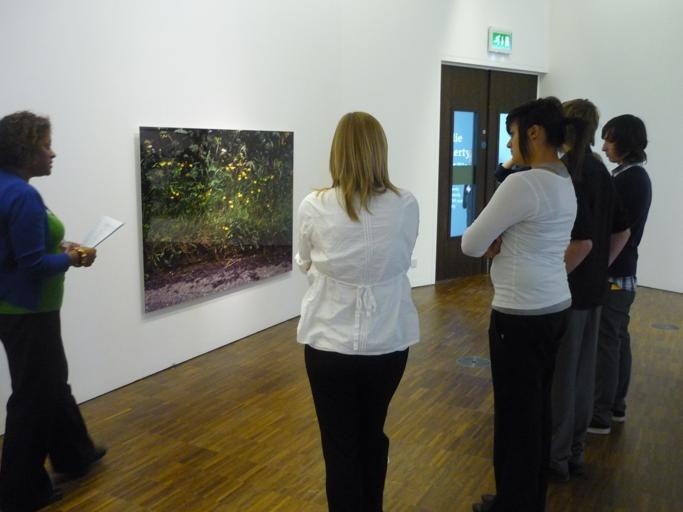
[70,246,86,267]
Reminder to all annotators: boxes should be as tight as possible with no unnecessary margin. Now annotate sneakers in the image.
[472,494,498,511]
[587,412,626,435]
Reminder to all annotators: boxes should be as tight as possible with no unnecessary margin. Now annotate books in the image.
[69,217,125,250]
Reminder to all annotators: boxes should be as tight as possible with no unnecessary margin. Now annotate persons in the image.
[549,100,631,482]
[587,114,652,435]
[0,110,107,511]
[491,149,529,181]
[295,111,421,511]
[461,96,579,511]
